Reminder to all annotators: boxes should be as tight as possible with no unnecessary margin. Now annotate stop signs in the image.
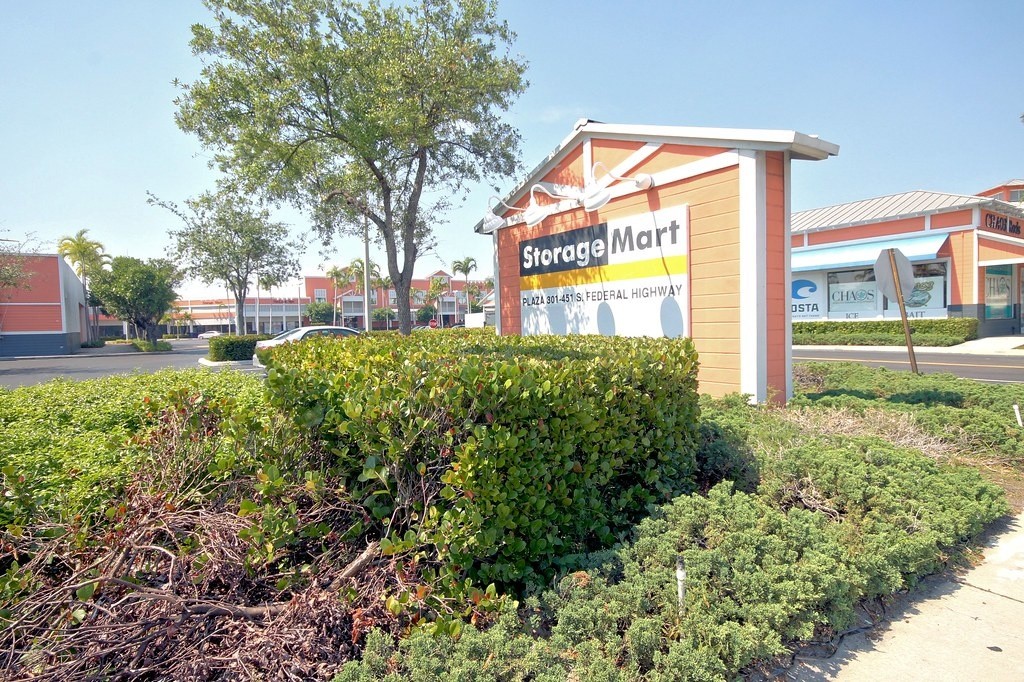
[429,319,437,328]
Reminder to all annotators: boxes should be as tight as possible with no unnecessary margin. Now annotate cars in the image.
[197,331,225,341]
[253,325,369,368]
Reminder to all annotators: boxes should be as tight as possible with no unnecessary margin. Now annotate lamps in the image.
[483,196,526,235]
[523,184,584,227]
[584,162,652,213]
